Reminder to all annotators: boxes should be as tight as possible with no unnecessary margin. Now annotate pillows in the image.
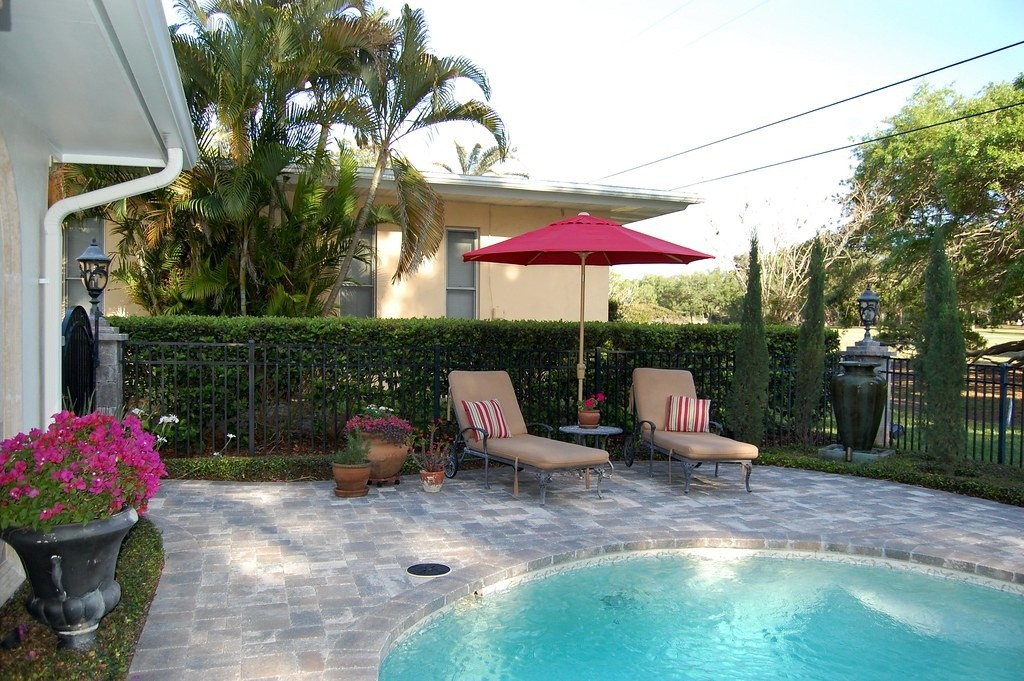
[461,397,514,442]
[665,396,711,433]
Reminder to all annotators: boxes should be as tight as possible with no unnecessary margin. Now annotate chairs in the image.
[446,370,610,504]
[625,368,758,494]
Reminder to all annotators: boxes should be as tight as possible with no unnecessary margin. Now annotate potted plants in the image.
[329,428,373,498]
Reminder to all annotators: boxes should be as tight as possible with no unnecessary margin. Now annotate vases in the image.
[0,506,139,653]
[358,433,408,483]
[418,469,446,492]
[577,411,600,429]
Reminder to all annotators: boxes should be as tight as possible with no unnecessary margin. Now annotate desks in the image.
[559,424,624,480]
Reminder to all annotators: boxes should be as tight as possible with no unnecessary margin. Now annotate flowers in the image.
[346,404,412,448]
[406,418,455,471]
[577,394,605,413]
[0,389,171,538]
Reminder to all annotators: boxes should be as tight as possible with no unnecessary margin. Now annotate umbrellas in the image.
[462,212,715,424]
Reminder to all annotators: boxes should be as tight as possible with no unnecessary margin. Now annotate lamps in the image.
[855,283,882,347]
[76,238,112,319]
